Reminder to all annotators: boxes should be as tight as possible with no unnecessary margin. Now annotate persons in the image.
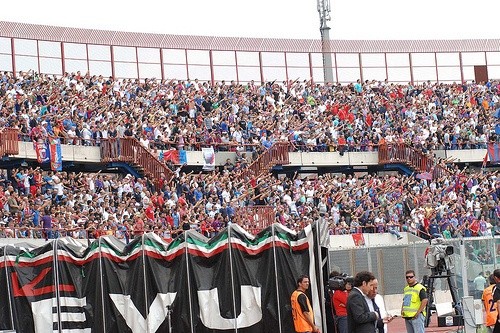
[0,68,500,265]
[473,269,500,333]
[291,274,320,333]
[401,270,429,333]
[326,271,395,333]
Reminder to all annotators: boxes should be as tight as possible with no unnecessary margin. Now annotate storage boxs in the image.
[437,315,463,327]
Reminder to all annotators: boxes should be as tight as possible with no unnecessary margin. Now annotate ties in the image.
[371,298,381,320]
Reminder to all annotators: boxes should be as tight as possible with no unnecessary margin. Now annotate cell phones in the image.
[393,315,398,317]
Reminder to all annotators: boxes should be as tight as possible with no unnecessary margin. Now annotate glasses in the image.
[406,276,414,279]
